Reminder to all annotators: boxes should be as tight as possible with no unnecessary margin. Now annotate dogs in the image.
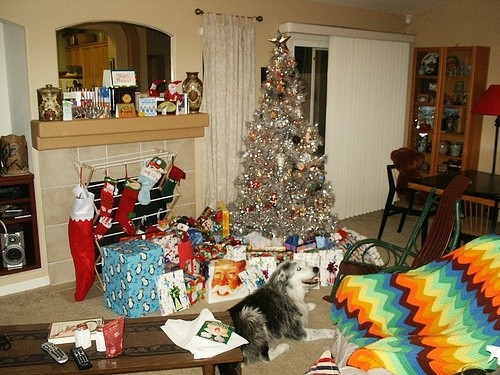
[217,259,336,375]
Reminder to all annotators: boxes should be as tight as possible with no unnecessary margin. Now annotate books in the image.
[47,317,104,344]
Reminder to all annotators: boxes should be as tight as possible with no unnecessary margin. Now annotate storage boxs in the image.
[100,239,165,319]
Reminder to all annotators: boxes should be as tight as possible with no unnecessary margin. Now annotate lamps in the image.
[470,84,500,175]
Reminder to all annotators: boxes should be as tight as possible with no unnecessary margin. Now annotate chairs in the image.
[377,147,438,246]
[322,174,472,302]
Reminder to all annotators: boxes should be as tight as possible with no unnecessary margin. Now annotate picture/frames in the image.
[108,58,114,70]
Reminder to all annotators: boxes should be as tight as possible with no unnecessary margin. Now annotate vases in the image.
[182,72,203,114]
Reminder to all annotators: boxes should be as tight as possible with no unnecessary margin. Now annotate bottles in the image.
[182,71,203,113]
[75,323,92,350]
[440,141,450,155]
[450,144,461,157]
[95,325,106,352]
[37,84,62,121]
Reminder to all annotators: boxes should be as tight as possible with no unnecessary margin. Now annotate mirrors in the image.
[56,22,172,98]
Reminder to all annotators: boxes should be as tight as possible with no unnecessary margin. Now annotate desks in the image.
[0,311,243,375]
[408,169,500,241]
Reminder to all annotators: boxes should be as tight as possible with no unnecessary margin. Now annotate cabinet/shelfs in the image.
[66,42,109,89]
[407,46,490,178]
[0,176,41,277]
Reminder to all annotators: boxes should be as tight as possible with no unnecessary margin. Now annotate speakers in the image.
[0,225,26,270]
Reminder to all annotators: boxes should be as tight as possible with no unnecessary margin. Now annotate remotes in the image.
[41,342,69,364]
[70,347,93,371]
